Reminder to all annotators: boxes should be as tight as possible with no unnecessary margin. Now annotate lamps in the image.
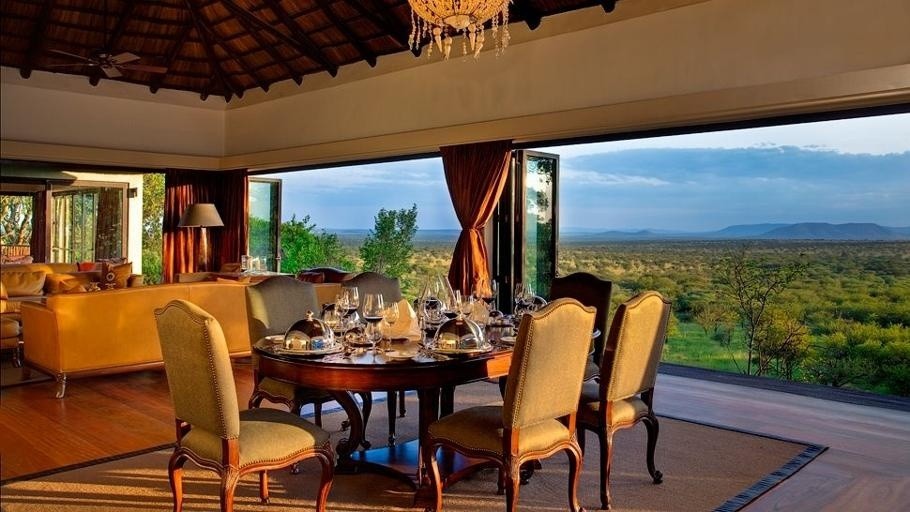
[176,203,224,275]
[409,0,509,56]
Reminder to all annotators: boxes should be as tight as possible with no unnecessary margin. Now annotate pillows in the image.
[100,260,131,288]
[1,271,45,296]
[45,273,90,293]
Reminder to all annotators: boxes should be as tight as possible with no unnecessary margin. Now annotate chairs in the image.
[549,271,609,369]
[150,300,334,512]
[582,289,672,510]
[244,274,344,474]
[341,273,405,450]
[422,298,596,511]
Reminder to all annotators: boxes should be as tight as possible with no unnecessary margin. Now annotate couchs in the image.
[18,277,251,398]
[0,261,145,367]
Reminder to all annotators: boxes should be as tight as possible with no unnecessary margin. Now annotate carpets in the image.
[0,389,830,512]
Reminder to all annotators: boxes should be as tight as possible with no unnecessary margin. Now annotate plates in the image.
[265,335,285,341]
[499,336,516,345]
[273,341,344,355]
[384,351,415,361]
[424,340,494,356]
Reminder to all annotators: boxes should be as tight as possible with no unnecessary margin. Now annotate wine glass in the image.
[341,286,359,320]
[472,279,497,311]
[334,293,349,344]
[443,290,462,319]
[383,302,400,352]
[362,293,384,350]
[514,282,534,314]
